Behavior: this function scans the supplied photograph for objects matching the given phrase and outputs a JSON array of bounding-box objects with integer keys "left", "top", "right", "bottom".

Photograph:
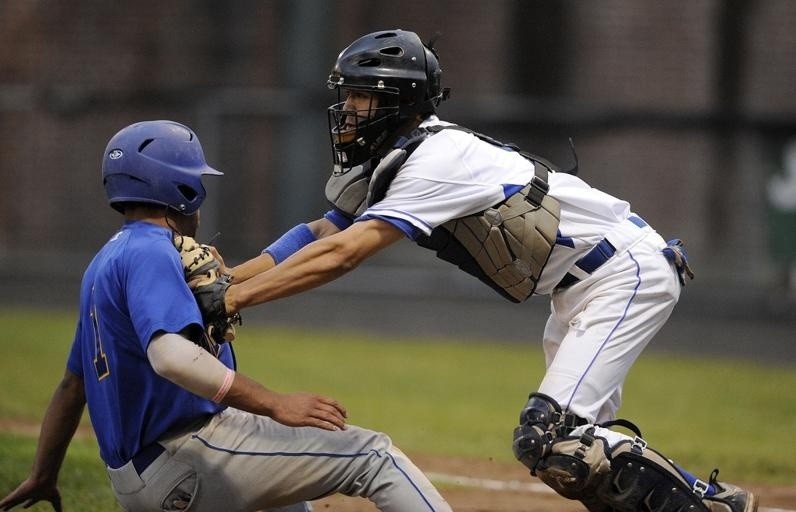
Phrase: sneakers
[{"left": 710, "top": 480, "right": 759, "bottom": 511}]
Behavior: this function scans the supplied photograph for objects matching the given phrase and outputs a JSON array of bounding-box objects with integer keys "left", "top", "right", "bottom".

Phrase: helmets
[
  {"left": 101, "top": 119, "right": 224, "bottom": 217},
  {"left": 326, "top": 28, "right": 441, "bottom": 177}
]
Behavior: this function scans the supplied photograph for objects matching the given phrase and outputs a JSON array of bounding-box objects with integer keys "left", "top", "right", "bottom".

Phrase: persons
[
  {"left": 172, "top": 27, "right": 759, "bottom": 511},
  {"left": 0, "top": 119, "right": 455, "bottom": 512}
]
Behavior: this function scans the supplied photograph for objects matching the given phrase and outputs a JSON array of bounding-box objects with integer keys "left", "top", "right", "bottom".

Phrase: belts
[
  {"left": 131, "top": 440, "right": 167, "bottom": 475},
  {"left": 554, "top": 216, "right": 646, "bottom": 287}
]
